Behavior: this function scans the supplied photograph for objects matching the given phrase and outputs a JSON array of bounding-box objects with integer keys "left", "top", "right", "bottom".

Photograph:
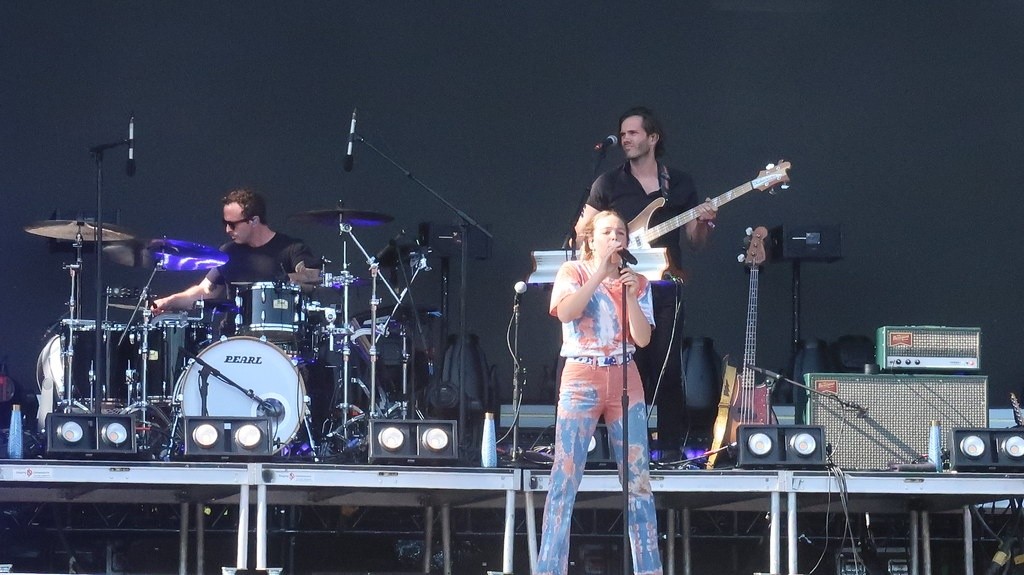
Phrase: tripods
[
  {"left": 54, "top": 229, "right": 185, "bottom": 459},
  {"left": 316, "top": 216, "right": 425, "bottom": 441}
]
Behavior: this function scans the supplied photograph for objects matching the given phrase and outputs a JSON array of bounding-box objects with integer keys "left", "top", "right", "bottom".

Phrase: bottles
[
  {"left": 928, "top": 419, "right": 943, "bottom": 473},
  {"left": 6, "top": 405, "right": 23, "bottom": 459},
  {"left": 481, "top": 413, "right": 498, "bottom": 468}
]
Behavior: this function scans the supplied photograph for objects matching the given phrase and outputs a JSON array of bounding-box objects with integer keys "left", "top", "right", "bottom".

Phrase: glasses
[{"left": 223, "top": 216, "right": 252, "bottom": 231}]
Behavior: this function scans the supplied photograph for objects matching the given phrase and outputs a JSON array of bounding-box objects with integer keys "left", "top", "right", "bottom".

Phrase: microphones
[
  {"left": 126, "top": 115, "right": 135, "bottom": 177},
  {"left": 594, "top": 135, "right": 618, "bottom": 150},
  {"left": 344, "top": 108, "right": 357, "bottom": 172},
  {"left": 618, "top": 248, "right": 638, "bottom": 265},
  {"left": 856, "top": 406, "right": 869, "bottom": 418},
  {"left": 373, "top": 229, "right": 404, "bottom": 263},
  {"left": 513, "top": 281, "right": 527, "bottom": 310},
  {"left": 254, "top": 395, "right": 281, "bottom": 417}
]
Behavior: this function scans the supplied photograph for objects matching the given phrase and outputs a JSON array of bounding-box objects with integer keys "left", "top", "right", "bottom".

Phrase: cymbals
[
  {"left": 285, "top": 208, "right": 396, "bottom": 227},
  {"left": 23, "top": 219, "right": 229, "bottom": 271},
  {"left": 303, "top": 277, "right": 381, "bottom": 287}
]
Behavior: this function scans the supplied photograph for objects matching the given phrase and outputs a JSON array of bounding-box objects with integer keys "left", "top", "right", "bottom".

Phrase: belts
[{"left": 570, "top": 354, "right": 634, "bottom": 366}]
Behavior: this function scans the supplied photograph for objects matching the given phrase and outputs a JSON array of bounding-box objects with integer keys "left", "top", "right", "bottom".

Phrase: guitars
[
  {"left": 704, "top": 226, "right": 780, "bottom": 469},
  {"left": 625, "top": 158, "right": 793, "bottom": 250}
]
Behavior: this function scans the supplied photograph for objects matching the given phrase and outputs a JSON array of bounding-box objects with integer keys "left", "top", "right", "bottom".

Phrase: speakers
[{"left": 803, "top": 373, "right": 990, "bottom": 471}]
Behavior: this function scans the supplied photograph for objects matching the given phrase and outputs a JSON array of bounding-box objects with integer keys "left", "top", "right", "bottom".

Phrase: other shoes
[{"left": 655, "top": 450, "right": 683, "bottom": 467}]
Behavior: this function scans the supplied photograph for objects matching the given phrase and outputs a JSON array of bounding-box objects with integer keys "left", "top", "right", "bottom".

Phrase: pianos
[{"left": 525, "top": 245, "right": 689, "bottom": 402}]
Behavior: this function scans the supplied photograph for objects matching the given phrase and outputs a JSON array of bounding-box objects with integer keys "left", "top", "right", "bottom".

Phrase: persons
[
  {"left": 150, "top": 189, "right": 322, "bottom": 457},
  {"left": 534, "top": 210, "right": 663, "bottom": 575},
  {"left": 569, "top": 106, "right": 718, "bottom": 462}
]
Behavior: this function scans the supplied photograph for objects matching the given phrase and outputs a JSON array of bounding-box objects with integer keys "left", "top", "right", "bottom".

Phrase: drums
[{"left": 36, "top": 280, "right": 357, "bottom": 459}]
